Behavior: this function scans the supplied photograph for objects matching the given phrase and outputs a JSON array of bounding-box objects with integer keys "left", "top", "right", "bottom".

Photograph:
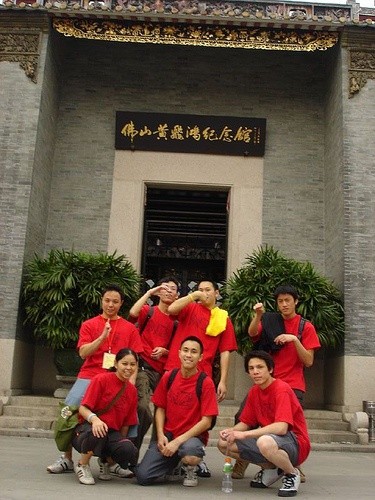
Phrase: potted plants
[{"left": 21, "top": 243, "right": 144, "bottom": 375}]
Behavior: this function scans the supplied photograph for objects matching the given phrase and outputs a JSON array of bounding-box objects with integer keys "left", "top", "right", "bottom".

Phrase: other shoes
[
  {"left": 297, "top": 466, "right": 305, "bottom": 482},
  {"left": 232, "top": 459, "right": 249, "bottom": 478}
]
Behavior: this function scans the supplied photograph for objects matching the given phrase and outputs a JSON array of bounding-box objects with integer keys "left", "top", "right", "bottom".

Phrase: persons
[
  {"left": 46, "top": 283, "right": 166, "bottom": 480},
  {"left": 74, "top": 348, "right": 139, "bottom": 486},
  {"left": 167, "top": 277, "right": 238, "bottom": 478},
  {"left": 134, "top": 335, "right": 219, "bottom": 487},
  {"left": 129, "top": 276, "right": 180, "bottom": 478},
  {"left": 231, "top": 284, "right": 321, "bottom": 477},
  {"left": 216, "top": 350, "right": 311, "bottom": 497}
]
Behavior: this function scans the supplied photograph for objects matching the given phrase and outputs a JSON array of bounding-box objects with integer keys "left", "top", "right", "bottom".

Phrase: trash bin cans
[{"left": 363, "top": 401, "right": 375, "bottom": 444}]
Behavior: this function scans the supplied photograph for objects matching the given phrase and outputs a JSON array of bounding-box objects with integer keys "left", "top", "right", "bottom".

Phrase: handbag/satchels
[
  {"left": 72, "top": 423, "right": 98, "bottom": 453},
  {"left": 54, "top": 400, "right": 81, "bottom": 451}
]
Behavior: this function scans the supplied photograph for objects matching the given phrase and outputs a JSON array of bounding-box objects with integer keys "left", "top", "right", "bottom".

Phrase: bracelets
[
  {"left": 189, "top": 293, "right": 194, "bottom": 302},
  {"left": 87, "top": 413, "right": 96, "bottom": 423}
]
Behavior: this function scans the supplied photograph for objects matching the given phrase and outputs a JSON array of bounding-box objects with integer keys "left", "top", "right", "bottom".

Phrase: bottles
[{"left": 221, "top": 458, "right": 234, "bottom": 494}]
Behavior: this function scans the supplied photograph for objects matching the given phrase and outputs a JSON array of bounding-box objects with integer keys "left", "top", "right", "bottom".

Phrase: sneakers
[
  {"left": 250, "top": 465, "right": 286, "bottom": 487},
  {"left": 76, "top": 461, "right": 95, "bottom": 484},
  {"left": 278, "top": 468, "right": 300, "bottom": 497},
  {"left": 197, "top": 461, "right": 211, "bottom": 477},
  {"left": 97, "top": 457, "right": 111, "bottom": 480},
  {"left": 109, "top": 463, "right": 133, "bottom": 477},
  {"left": 183, "top": 465, "right": 198, "bottom": 486},
  {"left": 47, "top": 456, "right": 75, "bottom": 473}
]
